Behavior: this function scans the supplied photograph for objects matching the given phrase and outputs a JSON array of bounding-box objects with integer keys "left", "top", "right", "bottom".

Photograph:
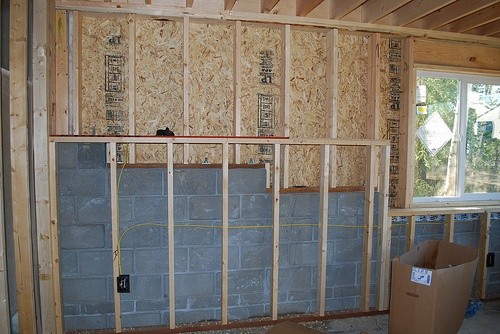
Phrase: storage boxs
[{"left": 385, "top": 239, "right": 480, "bottom": 334}]
